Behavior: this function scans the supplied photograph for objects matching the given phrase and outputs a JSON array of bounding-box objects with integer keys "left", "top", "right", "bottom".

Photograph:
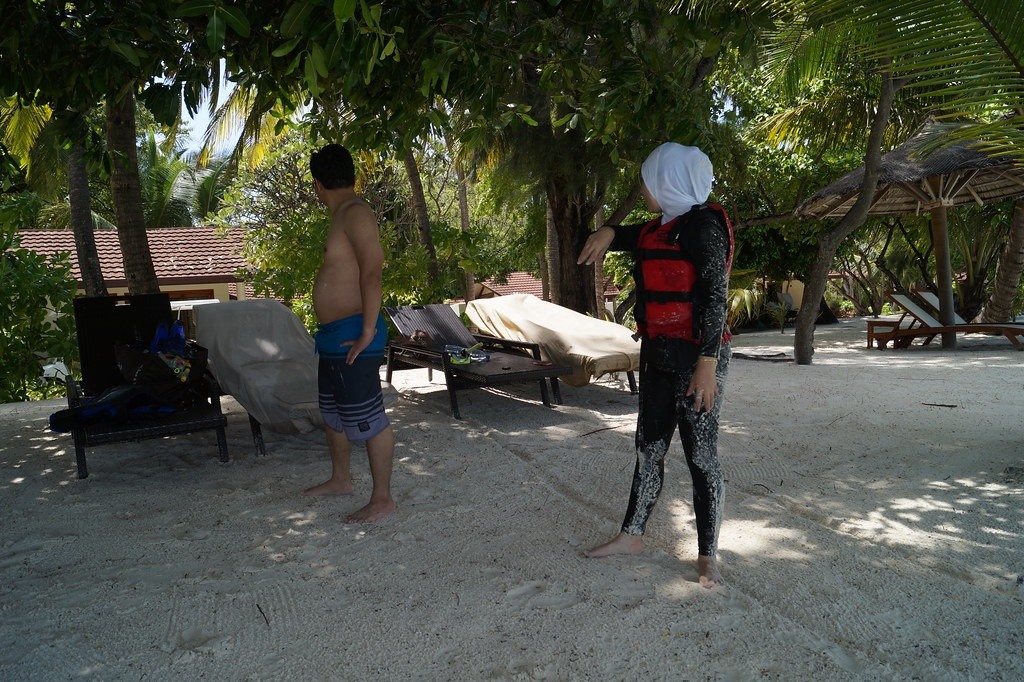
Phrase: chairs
[
  {"left": 191, "top": 299, "right": 398, "bottom": 457},
  {"left": 384, "top": 303, "right": 574, "bottom": 420},
  {"left": 463, "top": 292, "right": 642, "bottom": 405},
  {"left": 65, "top": 293, "right": 229, "bottom": 478},
  {"left": 874, "top": 292, "right": 1024, "bottom": 351},
  {"left": 912, "top": 288, "right": 1024, "bottom": 346}
]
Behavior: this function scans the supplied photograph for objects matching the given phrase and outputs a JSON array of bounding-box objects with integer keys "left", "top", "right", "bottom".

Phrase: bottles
[{"left": 445, "top": 345, "right": 491, "bottom": 362}]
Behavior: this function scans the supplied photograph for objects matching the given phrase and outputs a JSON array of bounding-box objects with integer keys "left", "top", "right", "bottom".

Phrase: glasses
[{"left": 312, "top": 179, "right": 316, "bottom": 187}]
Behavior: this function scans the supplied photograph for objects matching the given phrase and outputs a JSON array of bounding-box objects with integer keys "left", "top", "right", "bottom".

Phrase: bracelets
[{"left": 699, "top": 359, "right": 718, "bottom": 362}]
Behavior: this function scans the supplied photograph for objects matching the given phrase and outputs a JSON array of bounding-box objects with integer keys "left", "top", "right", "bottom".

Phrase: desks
[{"left": 866, "top": 320, "right": 901, "bottom": 349}]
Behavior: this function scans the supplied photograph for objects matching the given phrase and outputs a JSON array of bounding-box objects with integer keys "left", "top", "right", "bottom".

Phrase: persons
[
  {"left": 577, "top": 142, "right": 734, "bottom": 589},
  {"left": 301, "top": 143, "right": 394, "bottom": 523}
]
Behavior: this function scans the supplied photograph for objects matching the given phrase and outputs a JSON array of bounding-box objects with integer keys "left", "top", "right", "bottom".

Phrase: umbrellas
[{"left": 792, "top": 115, "right": 1024, "bottom": 351}]
